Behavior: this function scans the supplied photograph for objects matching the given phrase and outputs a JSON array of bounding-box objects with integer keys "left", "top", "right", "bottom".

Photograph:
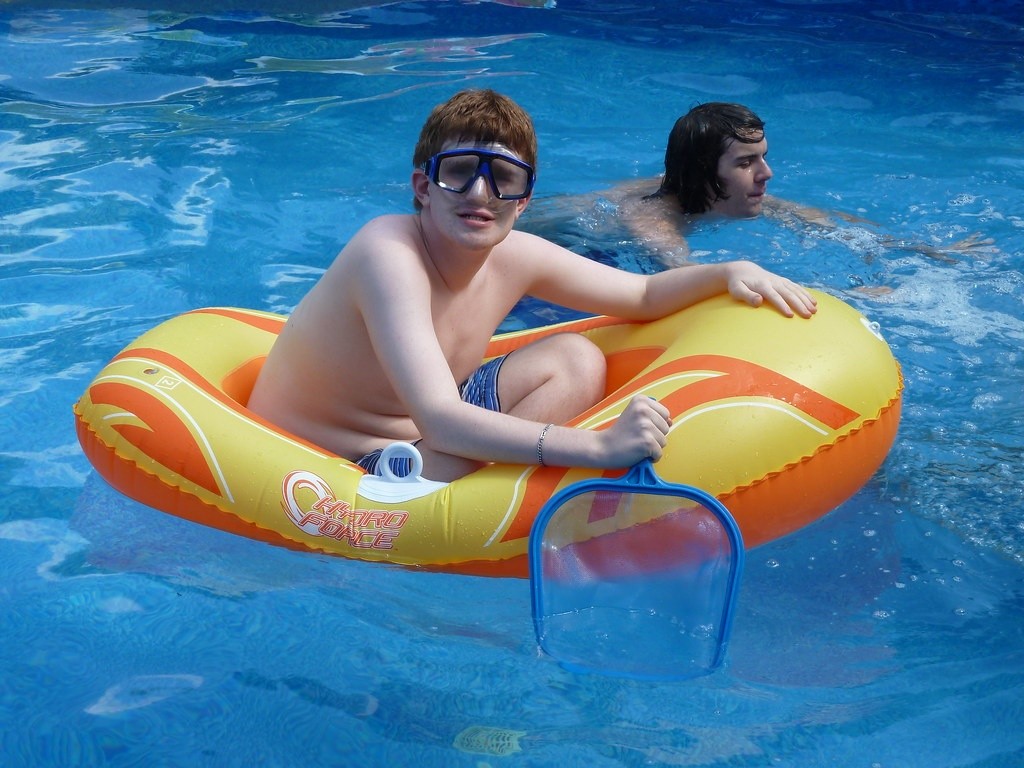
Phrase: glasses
[{"left": 418, "top": 149, "right": 534, "bottom": 200}]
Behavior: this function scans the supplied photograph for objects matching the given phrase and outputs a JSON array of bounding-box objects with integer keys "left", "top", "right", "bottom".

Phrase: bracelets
[{"left": 535, "top": 422, "right": 555, "bottom": 468}]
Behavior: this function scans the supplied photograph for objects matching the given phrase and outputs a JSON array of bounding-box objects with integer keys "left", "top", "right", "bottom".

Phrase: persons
[
  {"left": 510, "top": 102, "right": 999, "bottom": 300},
  {"left": 238, "top": 86, "right": 819, "bottom": 483}
]
